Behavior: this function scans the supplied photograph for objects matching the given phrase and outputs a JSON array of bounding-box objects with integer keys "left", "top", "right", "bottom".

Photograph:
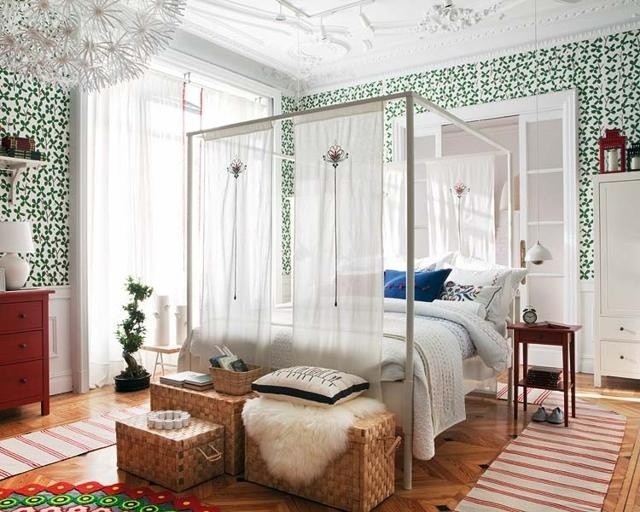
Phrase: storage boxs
[
  {"left": 116, "top": 411, "right": 225, "bottom": 493},
  {"left": 244, "top": 409, "right": 397, "bottom": 511},
  {"left": 149, "top": 381, "right": 258, "bottom": 477}
]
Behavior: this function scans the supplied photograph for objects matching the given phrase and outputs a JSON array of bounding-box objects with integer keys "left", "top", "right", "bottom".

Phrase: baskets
[{"left": 207, "top": 361, "right": 264, "bottom": 396}]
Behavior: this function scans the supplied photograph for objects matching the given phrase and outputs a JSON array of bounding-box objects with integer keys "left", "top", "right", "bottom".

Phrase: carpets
[
  {"left": 1, "top": 481, "right": 222, "bottom": 512},
  {"left": 0, "top": 401, "right": 150, "bottom": 481},
  {"left": 449, "top": 380, "right": 629, "bottom": 512}
]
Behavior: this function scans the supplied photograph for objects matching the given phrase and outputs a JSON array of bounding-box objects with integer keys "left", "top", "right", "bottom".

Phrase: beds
[{"left": 175, "top": 91, "right": 527, "bottom": 491}]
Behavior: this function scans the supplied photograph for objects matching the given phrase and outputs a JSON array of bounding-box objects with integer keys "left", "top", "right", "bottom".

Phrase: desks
[
  {"left": 505, "top": 321, "right": 583, "bottom": 427},
  {"left": 139, "top": 344, "right": 181, "bottom": 376}
]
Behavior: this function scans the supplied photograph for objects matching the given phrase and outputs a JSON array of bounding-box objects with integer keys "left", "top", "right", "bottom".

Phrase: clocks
[{"left": 523, "top": 305, "right": 537, "bottom": 324}]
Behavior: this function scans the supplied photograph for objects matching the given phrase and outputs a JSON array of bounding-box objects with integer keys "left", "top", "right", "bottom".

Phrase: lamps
[
  {"left": 523, "top": 1, "right": 554, "bottom": 266},
  {"left": 0, "top": 221, "right": 36, "bottom": 291}
]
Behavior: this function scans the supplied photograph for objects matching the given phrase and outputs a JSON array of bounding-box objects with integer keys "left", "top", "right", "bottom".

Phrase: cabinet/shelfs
[
  {"left": 0, "top": 288, "right": 55, "bottom": 417},
  {"left": 592, "top": 170, "right": 640, "bottom": 388}
]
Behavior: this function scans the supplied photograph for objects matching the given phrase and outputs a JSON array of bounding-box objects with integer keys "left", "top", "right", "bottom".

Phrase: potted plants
[{"left": 112, "top": 276, "right": 154, "bottom": 393}]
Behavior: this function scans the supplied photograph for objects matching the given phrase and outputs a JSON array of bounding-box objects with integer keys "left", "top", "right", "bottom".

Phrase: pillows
[
  {"left": 382, "top": 249, "right": 528, "bottom": 318},
  {"left": 251, "top": 366, "right": 369, "bottom": 408}
]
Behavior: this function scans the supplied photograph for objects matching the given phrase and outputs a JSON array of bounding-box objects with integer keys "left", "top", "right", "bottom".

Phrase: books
[
  {"left": 160, "top": 371, "right": 213, "bottom": 391},
  {"left": 209, "top": 354, "right": 248, "bottom": 372}
]
